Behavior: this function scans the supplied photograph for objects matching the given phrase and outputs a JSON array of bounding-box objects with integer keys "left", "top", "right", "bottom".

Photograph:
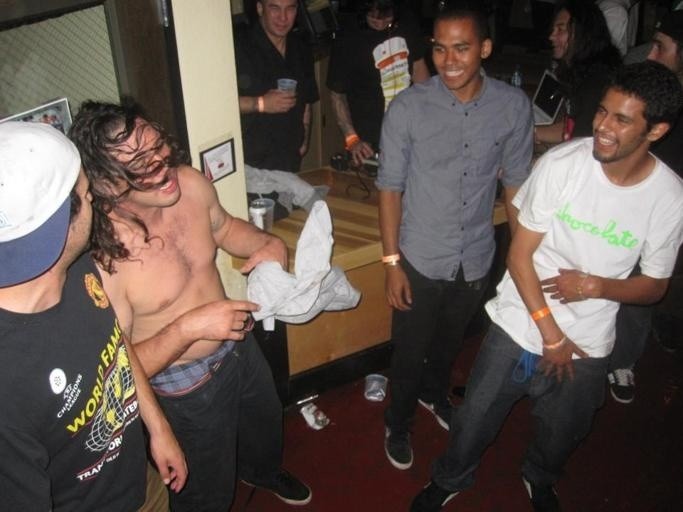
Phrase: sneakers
[
  {"left": 652, "top": 318, "right": 683, "bottom": 355},
  {"left": 409, "top": 476, "right": 461, "bottom": 512},
  {"left": 383, "top": 410, "right": 415, "bottom": 470},
  {"left": 608, "top": 368, "right": 637, "bottom": 403},
  {"left": 419, "top": 394, "right": 455, "bottom": 434},
  {"left": 522, "top": 471, "right": 562, "bottom": 512},
  {"left": 238, "top": 465, "right": 312, "bottom": 506}
]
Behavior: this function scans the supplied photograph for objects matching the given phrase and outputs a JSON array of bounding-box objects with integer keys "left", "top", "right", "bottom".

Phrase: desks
[{"left": 262, "top": 162, "right": 508, "bottom": 401}]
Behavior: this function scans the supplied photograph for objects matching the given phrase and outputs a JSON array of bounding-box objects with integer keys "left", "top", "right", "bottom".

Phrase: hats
[
  {"left": 656, "top": 10, "right": 683, "bottom": 43},
  {"left": 0, "top": 120, "right": 81, "bottom": 291}
]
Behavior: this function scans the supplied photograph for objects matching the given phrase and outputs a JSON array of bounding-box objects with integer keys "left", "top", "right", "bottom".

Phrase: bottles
[{"left": 510, "top": 72, "right": 522, "bottom": 88}]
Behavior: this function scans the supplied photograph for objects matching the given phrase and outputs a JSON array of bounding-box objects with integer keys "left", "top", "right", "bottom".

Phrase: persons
[
  {"left": 374, "top": 4, "right": 533, "bottom": 467},
  {"left": 230, "top": 2, "right": 682, "bottom": 225},
  {"left": 0, "top": 121, "right": 187, "bottom": 509},
  {"left": 66, "top": 97, "right": 311, "bottom": 510},
  {"left": 401, "top": 62, "right": 682, "bottom": 509}
]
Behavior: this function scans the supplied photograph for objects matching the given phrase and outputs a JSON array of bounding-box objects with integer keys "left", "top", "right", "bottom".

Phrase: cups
[
  {"left": 249, "top": 200, "right": 275, "bottom": 232},
  {"left": 277, "top": 78, "right": 298, "bottom": 92}
]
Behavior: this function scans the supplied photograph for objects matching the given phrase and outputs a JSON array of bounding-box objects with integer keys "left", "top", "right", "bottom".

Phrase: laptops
[{"left": 530, "top": 70, "right": 566, "bottom": 126}]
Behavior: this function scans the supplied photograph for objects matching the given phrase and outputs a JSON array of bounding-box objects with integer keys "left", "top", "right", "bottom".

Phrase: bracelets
[
  {"left": 380, "top": 253, "right": 402, "bottom": 265},
  {"left": 539, "top": 334, "right": 566, "bottom": 353},
  {"left": 574, "top": 271, "right": 594, "bottom": 302},
  {"left": 528, "top": 304, "right": 552, "bottom": 325}
]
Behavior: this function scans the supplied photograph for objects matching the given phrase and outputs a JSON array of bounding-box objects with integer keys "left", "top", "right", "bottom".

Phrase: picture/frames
[
  {"left": 198, "top": 137, "right": 236, "bottom": 184},
  {"left": 0, "top": 97, "right": 72, "bottom": 136}
]
